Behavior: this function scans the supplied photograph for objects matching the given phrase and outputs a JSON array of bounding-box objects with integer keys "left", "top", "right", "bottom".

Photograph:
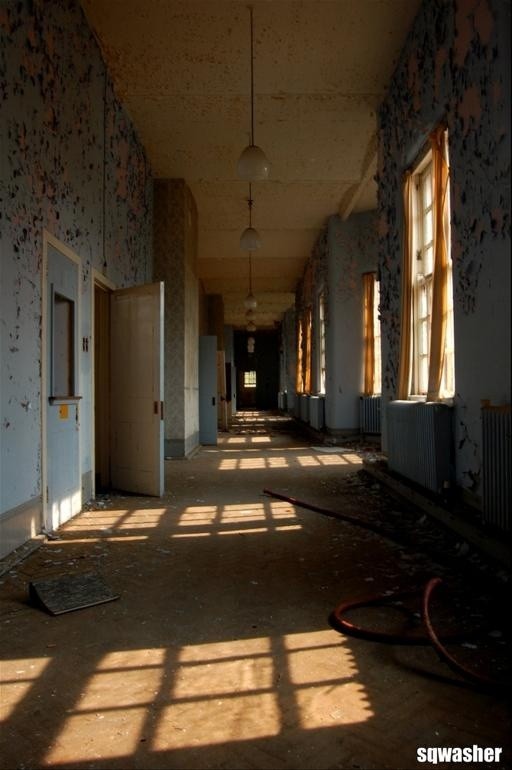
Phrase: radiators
[
  {"left": 385, "top": 401, "right": 455, "bottom": 498},
  {"left": 277, "top": 392, "right": 323, "bottom": 432},
  {"left": 481, "top": 404, "right": 512, "bottom": 539},
  {"left": 359, "top": 396, "right": 382, "bottom": 443}
]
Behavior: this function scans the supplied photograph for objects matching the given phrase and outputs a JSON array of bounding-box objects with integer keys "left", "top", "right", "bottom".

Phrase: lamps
[{"left": 234, "top": 1, "right": 273, "bottom": 352}]
